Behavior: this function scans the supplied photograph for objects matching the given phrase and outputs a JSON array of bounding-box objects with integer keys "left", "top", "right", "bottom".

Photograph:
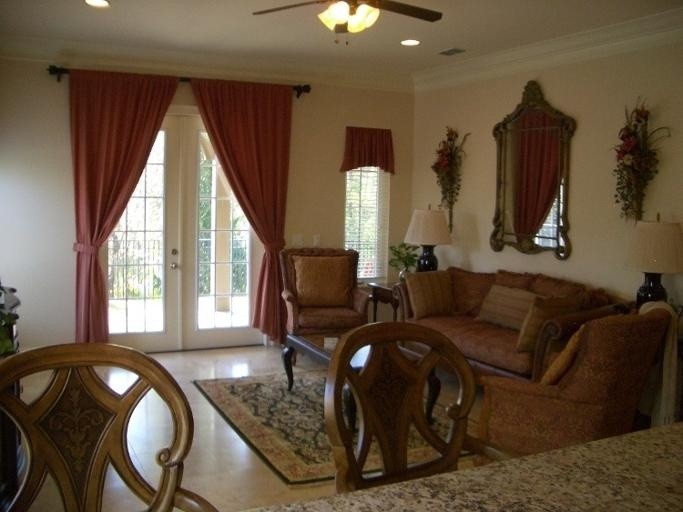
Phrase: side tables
[{"left": 367, "top": 279, "right": 399, "bottom": 322}]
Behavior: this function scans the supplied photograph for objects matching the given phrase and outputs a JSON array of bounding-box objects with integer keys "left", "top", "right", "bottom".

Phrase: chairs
[{"left": 0, "top": 340, "right": 220, "bottom": 511}]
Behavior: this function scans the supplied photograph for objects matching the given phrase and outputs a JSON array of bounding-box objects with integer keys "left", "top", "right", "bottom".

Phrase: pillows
[
  {"left": 528, "top": 271, "right": 585, "bottom": 296},
  {"left": 446, "top": 265, "right": 495, "bottom": 317},
  {"left": 496, "top": 267, "right": 539, "bottom": 289},
  {"left": 514, "top": 295, "right": 584, "bottom": 352},
  {"left": 287, "top": 252, "right": 356, "bottom": 310},
  {"left": 471, "top": 284, "right": 548, "bottom": 332},
  {"left": 404, "top": 269, "right": 454, "bottom": 321}
]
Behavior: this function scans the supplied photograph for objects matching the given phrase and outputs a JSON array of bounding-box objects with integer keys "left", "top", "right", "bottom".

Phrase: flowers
[
  {"left": 427, "top": 124, "right": 473, "bottom": 234},
  {"left": 605, "top": 93, "right": 672, "bottom": 230}
]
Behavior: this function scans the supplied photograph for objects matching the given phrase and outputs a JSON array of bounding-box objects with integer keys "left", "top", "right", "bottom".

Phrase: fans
[{"left": 249, "top": 1, "right": 444, "bottom": 25}]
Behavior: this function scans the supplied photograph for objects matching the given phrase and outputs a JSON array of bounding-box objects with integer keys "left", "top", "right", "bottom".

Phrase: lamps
[
  {"left": 403, "top": 203, "right": 452, "bottom": 273},
  {"left": 315, "top": 1, "right": 380, "bottom": 49},
  {"left": 621, "top": 212, "right": 683, "bottom": 314}
]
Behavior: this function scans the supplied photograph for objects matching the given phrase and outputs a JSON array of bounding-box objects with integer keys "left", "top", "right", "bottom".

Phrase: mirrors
[{"left": 488, "top": 80, "right": 577, "bottom": 261}]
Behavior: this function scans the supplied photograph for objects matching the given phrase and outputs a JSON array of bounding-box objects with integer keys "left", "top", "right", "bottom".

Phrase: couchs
[
  {"left": 276, "top": 246, "right": 374, "bottom": 367},
  {"left": 472, "top": 306, "right": 671, "bottom": 467},
  {"left": 389, "top": 264, "right": 637, "bottom": 383}
]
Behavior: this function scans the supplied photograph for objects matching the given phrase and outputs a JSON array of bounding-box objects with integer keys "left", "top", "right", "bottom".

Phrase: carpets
[{"left": 189, "top": 366, "right": 480, "bottom": 491}]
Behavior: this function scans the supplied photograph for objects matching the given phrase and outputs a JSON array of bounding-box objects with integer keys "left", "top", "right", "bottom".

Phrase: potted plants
[{"left": 387, "top": 241, "right": 420, "bottom": 282}]
